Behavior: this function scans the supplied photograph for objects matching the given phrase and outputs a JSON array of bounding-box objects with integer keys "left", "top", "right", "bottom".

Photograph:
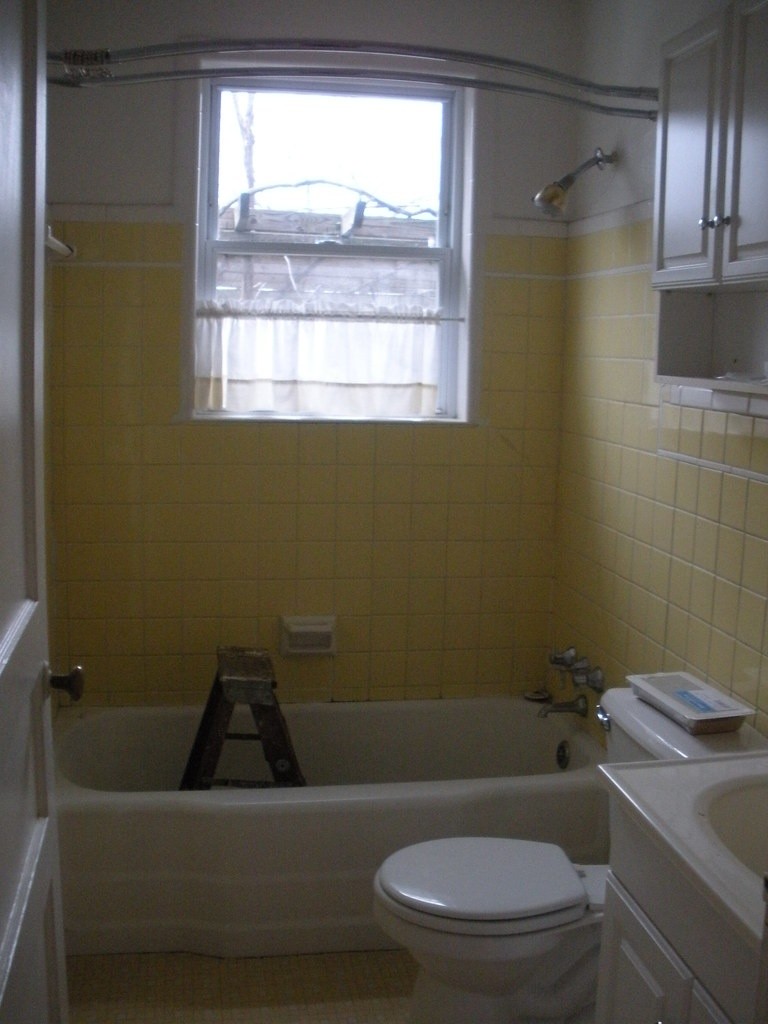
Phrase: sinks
[{"left": 688, "top": 769, "right": 768, "bottom": 910}]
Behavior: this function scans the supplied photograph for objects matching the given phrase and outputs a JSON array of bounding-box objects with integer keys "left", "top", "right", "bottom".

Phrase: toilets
[{"left": 371, "top": 684, "right": 767, "bottom": 1023}]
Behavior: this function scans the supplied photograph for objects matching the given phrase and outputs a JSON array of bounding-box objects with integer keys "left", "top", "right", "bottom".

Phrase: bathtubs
[{"left": 54, "top": 693, "right": 607, "bottom": 961}]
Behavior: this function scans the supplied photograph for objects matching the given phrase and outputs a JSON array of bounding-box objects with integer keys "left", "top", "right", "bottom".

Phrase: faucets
[{"left": 537, "top": 695, "right": 588, "bottom": 719}]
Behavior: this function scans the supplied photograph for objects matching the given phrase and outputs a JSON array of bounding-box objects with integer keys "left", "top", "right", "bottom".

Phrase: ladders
[{"left": 174, "top": 646, "right": 308, "bottom": 791}]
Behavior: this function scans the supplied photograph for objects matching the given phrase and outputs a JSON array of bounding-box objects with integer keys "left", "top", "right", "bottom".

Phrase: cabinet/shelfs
[
  {"left": 649, "top": 0, "right": 767, "bottom": 397},
  {"left": 592, "top": 790, "right": 768, "bottom": 1024}
]
